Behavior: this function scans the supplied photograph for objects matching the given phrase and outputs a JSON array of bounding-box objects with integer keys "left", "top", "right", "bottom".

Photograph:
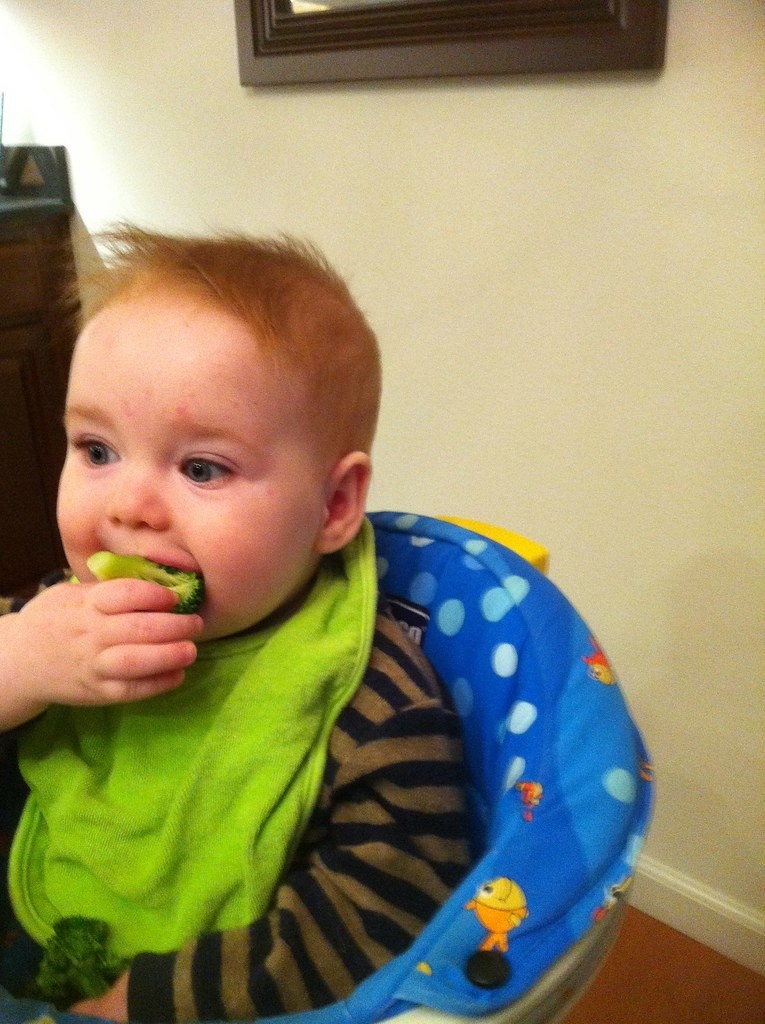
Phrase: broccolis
[
  {"left": 39, "top": 916, "right": 117, "bottom": 1003},
  {"left": 68, "top": 550, "right": 205, "bottom": 615}
]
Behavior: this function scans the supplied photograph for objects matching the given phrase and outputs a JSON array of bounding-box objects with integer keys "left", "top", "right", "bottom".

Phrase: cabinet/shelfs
[{"left": 0, "top": 182, "right": 82, "bottom": 597}]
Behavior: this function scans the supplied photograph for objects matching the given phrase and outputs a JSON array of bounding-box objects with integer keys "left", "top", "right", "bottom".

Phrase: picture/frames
[{"left": 234, "top": 0, "right": 668, "bottom": 86}]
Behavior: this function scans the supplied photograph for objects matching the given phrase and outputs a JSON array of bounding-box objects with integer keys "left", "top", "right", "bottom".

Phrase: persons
[{"left": 0, "top": 232, "right": 476, "bottom": 1024}]
